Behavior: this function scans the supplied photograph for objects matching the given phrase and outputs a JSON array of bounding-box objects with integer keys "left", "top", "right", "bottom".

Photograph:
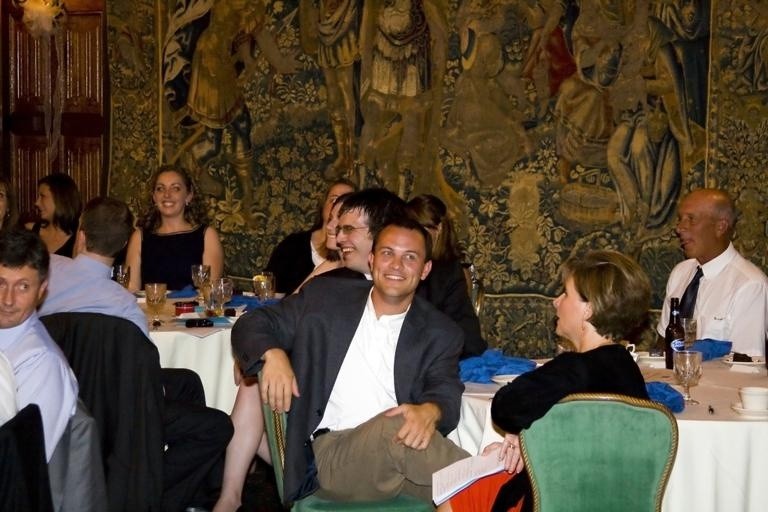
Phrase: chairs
[
  {"left": 256, "top": 370, "right": 433, "bottom": 512},
  {"left": 0, "top": 403, "right": 55, "bottom": 512},
  {"left": 37, "top": 312, "right": 235, "bottom": 512},
  {"left": 517, "top": 393, "right": 677, "bottom": 512}
]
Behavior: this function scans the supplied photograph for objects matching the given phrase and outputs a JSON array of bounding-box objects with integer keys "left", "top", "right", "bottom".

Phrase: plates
[
  {"left": 721, "top": 359, "right": 766, "bottom": 365},
  {"left": 228, "top": 316, "right": 239, "bottom": 321},
  {"left": 640, "top": 355, "right": 666, "bottom": 365},
  {"left": 731, "top": 405, "right": 768, "bottom": 416},
  {"left": 490, "top": 374, "right": 519, "bottom": 381}
]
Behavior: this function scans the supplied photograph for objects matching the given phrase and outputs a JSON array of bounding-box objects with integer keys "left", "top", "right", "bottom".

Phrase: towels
[
  {"left": 167, "top": 285, "right": 199, "bottom": 298},
  {"left": 224, "top": 296, "right": 257, "bottom": 306},
  {"left": 687, "top": 338, "right": 732, "bottom": 362},
  {"left": 481, "top": 350, "right": 537, "bottom": 375},
  {"left": 458, "top": 357, "right": 498, "bottom": 383},
  {"left": 645, "top": 381, "right": 685, "bottom": 413},
  {"left": 243, "top": 299, "right": 278, "bottom": 311}
]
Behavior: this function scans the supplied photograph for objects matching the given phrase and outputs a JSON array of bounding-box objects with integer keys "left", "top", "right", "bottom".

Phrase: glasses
[{"left": 335, "top": 225, "right": 369, "bottom": 235}]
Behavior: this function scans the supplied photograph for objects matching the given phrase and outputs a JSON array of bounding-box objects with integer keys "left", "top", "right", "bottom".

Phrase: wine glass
[
  {"left": 252, "top": 272, "right": 272, "bottom": 300},
  {"left": 146, "top": 283, "right": 166, "bottom": 326},
  {"left": 192, "top": 265, "right": 211, "bottom": 302},
  {"left": 116, "top": 265, "right": 130, "bottom": 287},
  {"left": 681, "top": 318, "right": 697, "bottom": 349},
  {"left": 673, "top": 352, "right": 703, "bottom": 406},
  {"left": 206, "top": 284, "right": 223, "bottom": 317}
]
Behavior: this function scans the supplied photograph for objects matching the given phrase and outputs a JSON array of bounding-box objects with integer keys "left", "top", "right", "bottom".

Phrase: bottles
[{"left": 664, "top": 295, "right": 687, "bottom": 370}]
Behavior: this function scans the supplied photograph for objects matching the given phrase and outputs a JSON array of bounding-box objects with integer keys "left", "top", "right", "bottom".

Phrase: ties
[{"left": 679, "top": 266, "right": 704, "bottom": 318}]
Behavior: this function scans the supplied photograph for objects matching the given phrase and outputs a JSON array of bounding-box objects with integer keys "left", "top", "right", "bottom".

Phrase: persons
[
  {"left": 654, "top": 188, "right": 765, "bottom": 356},
  {"left": 18, "top": 172, "right": 82, "bottom": 255},
  {"left": 0, "top": 224, "right": 80, "bottom": 467},
  {"left": 230, "top": 215, "right": 475, "bottom": 512},
  {"left": 296, "top": 189, "right": 408, "bottom": 295},
  {"left": 32, "top": 195, "right": 150, "bottom": 350},
  {"left": 262, "top": 180, "right": 356, "bottom": 292},
  {"left": 124, "top": 167, "right": 222, "bottom": 306},
  {"left": 433, "top": 251, "right": 648, "bottom": 512},
  {"left": 212, "top": 189, "right": 355, "bottom": 511},
  {"left": 404, "top": 195, "right": 487, "bottom": 358}
]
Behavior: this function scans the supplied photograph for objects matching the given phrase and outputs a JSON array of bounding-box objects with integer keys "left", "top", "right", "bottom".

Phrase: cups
[
  {"left": 740, "top": 387, "right": 768, "bottom": 411},
  {"left": 215, "top": 278, "right": 233, "bottom": 303}
]
